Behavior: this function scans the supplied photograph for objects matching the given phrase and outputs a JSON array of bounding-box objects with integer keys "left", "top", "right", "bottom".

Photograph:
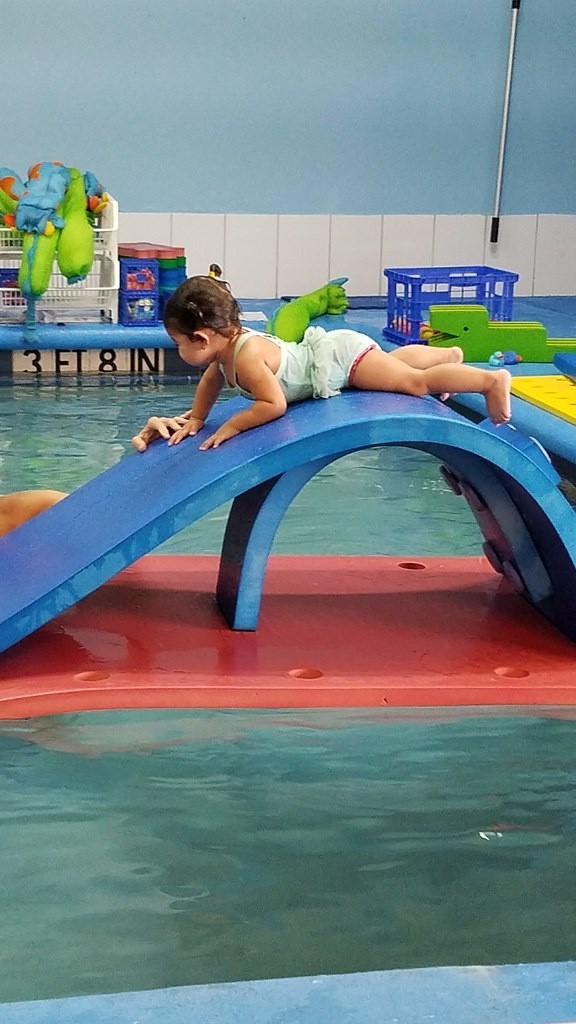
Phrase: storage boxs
[
  {"left": 382, "top": 265, "right": 519, "bottom": 346},
  {"left": 238, "top": 311, "right": 269, "bottom": 333},
  {"left": 0, "top": 192, "right": 161, "bottom": 328}
]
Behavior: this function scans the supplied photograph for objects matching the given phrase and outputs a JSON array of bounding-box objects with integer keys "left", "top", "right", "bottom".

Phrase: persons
[{"left": 162, "top": 264, "right": 512, "bottom": 451}]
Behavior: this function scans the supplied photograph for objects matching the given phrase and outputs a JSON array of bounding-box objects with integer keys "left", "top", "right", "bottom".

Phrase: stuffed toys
[
  {"left": 265, "top": 277, "right": 350, "bottom": 344},
  {"left": 0, "top": 161, "right": 110, "bottom": 299}
]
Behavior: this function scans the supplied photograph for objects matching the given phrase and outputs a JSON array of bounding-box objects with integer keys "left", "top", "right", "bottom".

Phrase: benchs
[{"left": 0, "top": 321, "right": 199, "bottom": 373}]
